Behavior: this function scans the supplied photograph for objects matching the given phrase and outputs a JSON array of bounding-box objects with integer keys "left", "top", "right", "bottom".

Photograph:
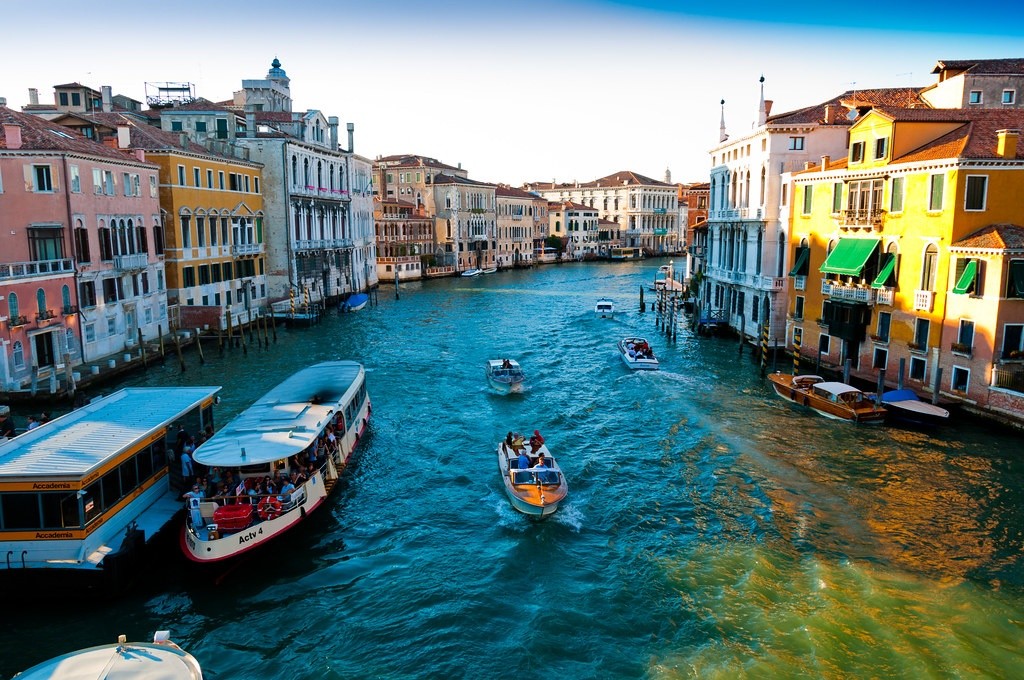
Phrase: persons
[
  {"left": 501, "top": 358, "right": 513, "bottom": 371},
  {"left": 533, "top": 452, "right": 551, "bottom": 480},
  {"left": 507, "top": 430, "right": 544, "bottom": 455},
  {"left": 628, "top": 340, "right": 653, "bottom": 358},
  {"left": 28, "top": 415, "right": 40, "bottom": 429},
  {"left": 518, "top": 449, "right": 530, "bottom": 469},
  {"left": 168, "top": 413, "right": 343, "bottom": 499},
  {"left": 40, "top": 412, "right": 51, "bottom": 424},
  {"left": 0, "top": 415, "right": 15, "bottom": 437}
]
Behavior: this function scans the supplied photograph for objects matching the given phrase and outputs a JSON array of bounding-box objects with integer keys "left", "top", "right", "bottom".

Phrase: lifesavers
[{"left": 257, "top": 497, "right": 282, "bottom": 520}]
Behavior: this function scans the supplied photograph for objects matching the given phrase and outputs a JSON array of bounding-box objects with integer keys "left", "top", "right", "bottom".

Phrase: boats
[
  {"left": 481, "top": 268, "right": 498, "bottom": 274},
  {"left": 11, "top": 629, "right": 202, "bottom": 680},
  {"left": 497, "top": 430, "right": 569, "bottom": 518},
  {"left": 610, "top": 248, "right": 643, "bottom": 259},
  {"left": 486, "top": 359, "right": 523, "bottom": 392},
  {"left": 861, "top": 388, "right": 950, "bottom": 419},
  {"left": 595, "top": 300, "right": 614, "bottom": 319},
  {"left": 178, "top": 359, "right": 373, "bottom": 564},
  {"left": 649, "top": 277, "right": 685, "bottom": 291},
  {"left": 339, "top": 293, "right": 369, "bottom": 311},
  {"left": 766, "top": 373, "right": 887, "bottom": 424},
  {"left": 462, "top": 268, "right": 482, "bottom": 276},
  {"left": 618, "top": 337, "right": 660, "bottom": 370},
  {"left": 0, "top": 383, "right": 220, "bottom": 569}
]
